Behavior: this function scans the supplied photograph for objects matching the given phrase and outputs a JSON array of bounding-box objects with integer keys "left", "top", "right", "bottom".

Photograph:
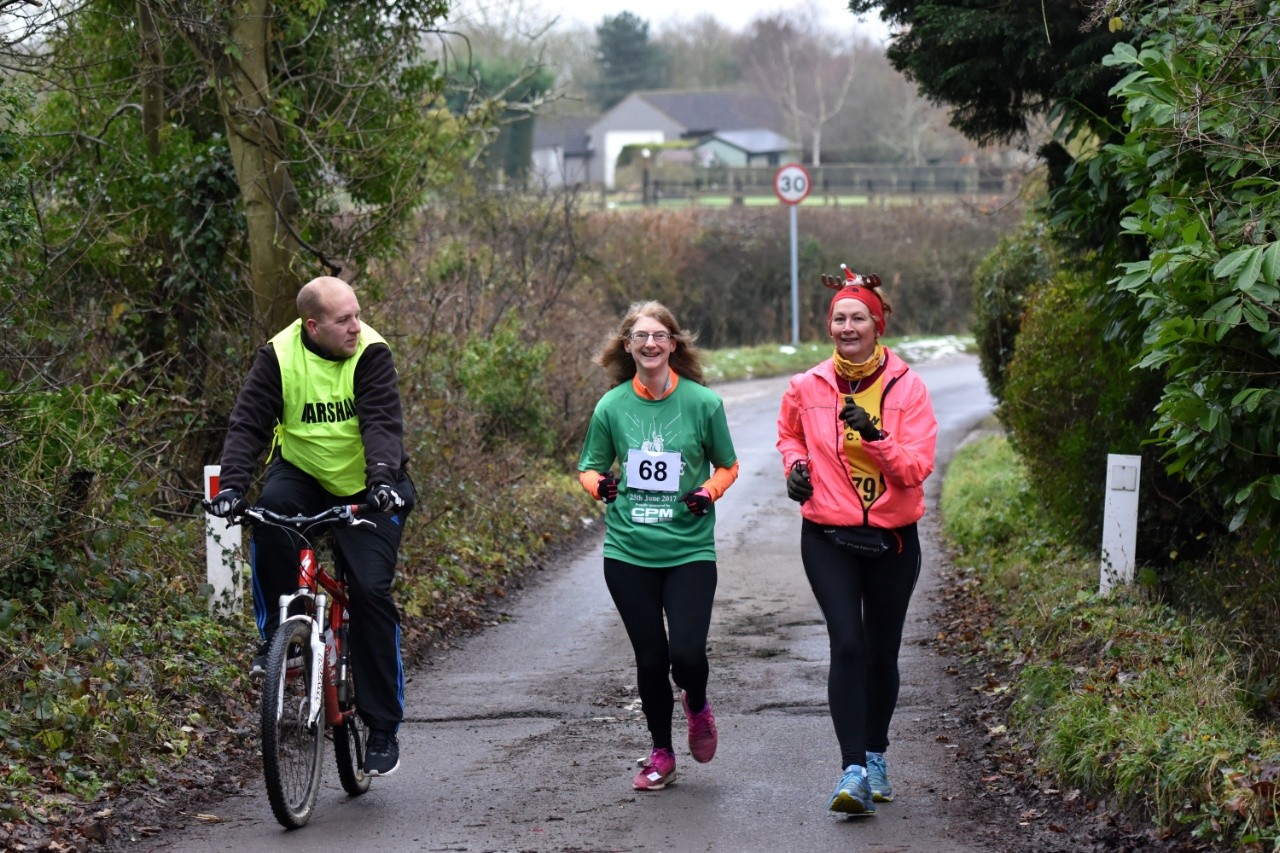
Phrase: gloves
[
  {"left": 676, "top": 486, "right": 711, "bottom": 517},
  {"left": 593, "top": 471, "right": 620, "bottom": 504},
  {"left": 369, "top": 482, "right": 408, "bottom": 518},
  {"left": 838, "top": 396, "right": 880, "bottom": 442},
  {"left": 787, "top": 462, "right": 816, "bottom": 502},
  {"left": 209, "top": 488, "right": 250, "bottom": 516}
]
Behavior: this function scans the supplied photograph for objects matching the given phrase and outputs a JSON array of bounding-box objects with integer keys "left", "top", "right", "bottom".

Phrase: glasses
[
  {"left": 795, "top": 459, "right": 812, "bottom": 477},
  {"left": 627, "top": 330, "right": 675, "bottom": 344}
]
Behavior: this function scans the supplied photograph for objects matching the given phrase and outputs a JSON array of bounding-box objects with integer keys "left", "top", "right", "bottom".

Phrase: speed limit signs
[{"left": 772, "top": 160, "right": 811, "bottom": 205}]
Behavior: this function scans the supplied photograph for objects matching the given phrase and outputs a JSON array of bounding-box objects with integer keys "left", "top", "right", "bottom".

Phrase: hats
[{"left": 820, "top": 263, "right": 887, "bottom": 336}]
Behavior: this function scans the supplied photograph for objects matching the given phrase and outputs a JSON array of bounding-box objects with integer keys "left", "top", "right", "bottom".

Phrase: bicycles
[{"left": 204, "top": 487, "right": 382, "bottom": 831}]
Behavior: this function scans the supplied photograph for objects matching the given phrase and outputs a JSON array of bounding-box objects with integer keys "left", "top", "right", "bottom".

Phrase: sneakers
[
  {"left": 829, "top": 764, "right": 879, "bottom": 815},
  {"left": 363, "top": 732, "right": 403, "bottom": 781},
  {"left": 680, "top": 689, "right": 718, "bottom": 764},
  {"left": 633, "top": 746, "right": 676, "bottom": 790},
  {"left": 865, "top": 750, "right": 895, "bottom": 802},
  {"left": 248, "top": 642, "right": 309, "bottom": 677}
]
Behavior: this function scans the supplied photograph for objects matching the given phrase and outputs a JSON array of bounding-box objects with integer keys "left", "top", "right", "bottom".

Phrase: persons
[
  {"left": 776, "top": 260, "right": 937, "bottom": 822},
  {"left": 212, "top": 275, "right": 414, "bottom": 776},
  {"left": 579, "top": 301, "right": 738, "bottom": 790}
]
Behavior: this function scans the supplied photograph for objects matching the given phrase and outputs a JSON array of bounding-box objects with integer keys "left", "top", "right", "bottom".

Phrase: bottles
[{"left": 320, "top": 617, "right": 338, "bottom": 688}]
